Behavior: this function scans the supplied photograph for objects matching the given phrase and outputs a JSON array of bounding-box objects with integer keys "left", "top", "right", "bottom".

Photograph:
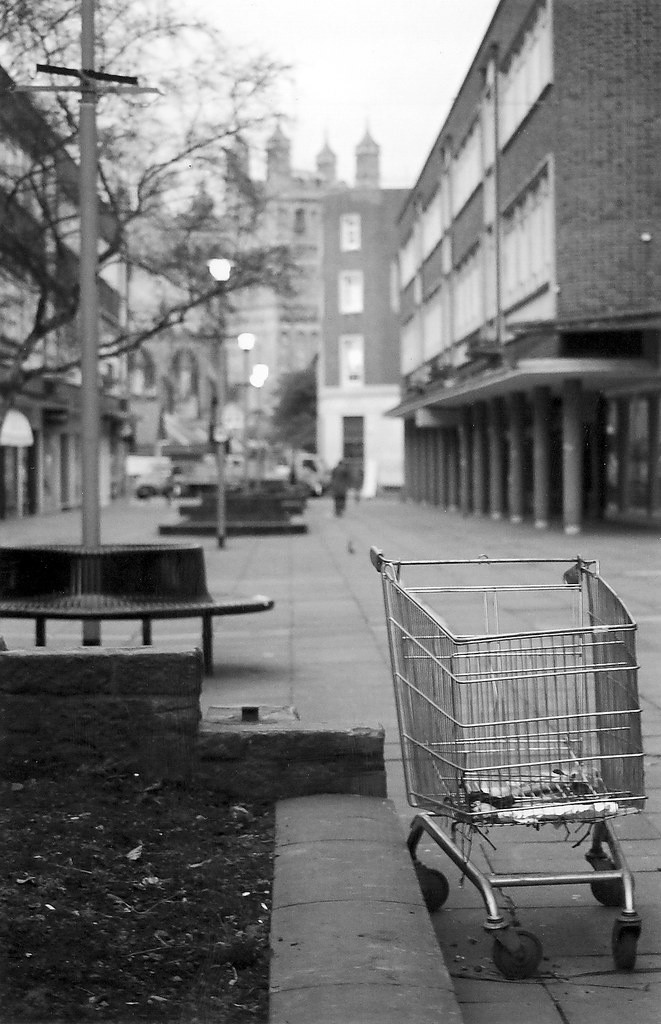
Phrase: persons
[{"left": 333, "top": 461, "right": 351, "bottom": 516}]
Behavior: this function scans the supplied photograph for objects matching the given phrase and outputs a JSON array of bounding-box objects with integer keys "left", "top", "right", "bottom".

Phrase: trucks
[{"left": 190, "top": 447, "right": 332, "bottom": 498}]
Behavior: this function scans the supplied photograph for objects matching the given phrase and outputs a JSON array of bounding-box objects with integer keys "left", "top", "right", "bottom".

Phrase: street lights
[{"left": 209, "top": 259, "right": 234, "bottom": 548}]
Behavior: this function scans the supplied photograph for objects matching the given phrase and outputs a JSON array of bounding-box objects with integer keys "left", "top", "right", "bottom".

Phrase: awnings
[{"left": 0, "top": 409, "right": 33, "bottom": 447}]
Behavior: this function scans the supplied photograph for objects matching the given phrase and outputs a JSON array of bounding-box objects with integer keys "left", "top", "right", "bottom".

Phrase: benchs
[{"left": 0, "top": 543, "right": 276, "bottom": 677}]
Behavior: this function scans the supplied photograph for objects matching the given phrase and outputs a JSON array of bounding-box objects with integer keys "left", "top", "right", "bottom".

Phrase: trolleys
[{"left": 368, "top": 545, "right": 647, "bottom": 980}]
